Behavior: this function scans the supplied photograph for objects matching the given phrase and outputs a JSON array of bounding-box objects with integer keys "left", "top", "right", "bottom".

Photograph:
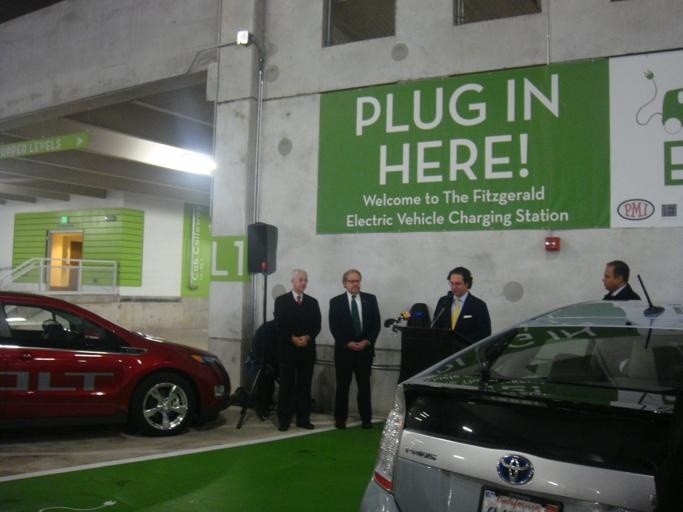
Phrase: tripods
[{"left": 237, "top": 283, "right": 280, "bottom": 427}]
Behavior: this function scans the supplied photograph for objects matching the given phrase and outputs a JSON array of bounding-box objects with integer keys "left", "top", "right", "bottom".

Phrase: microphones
[{"left": 401, "top": 311, "right": 423, "bottom": 321}]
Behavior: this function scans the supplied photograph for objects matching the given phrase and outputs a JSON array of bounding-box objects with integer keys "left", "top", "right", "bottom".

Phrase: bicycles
[{"left": 235, "top": 357, "right": 275, "bottom": 434}]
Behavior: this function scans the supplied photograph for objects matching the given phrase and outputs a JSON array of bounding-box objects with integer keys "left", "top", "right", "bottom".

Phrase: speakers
[{"left": 247, "top": 222, "right": 279, "bottom": 275}]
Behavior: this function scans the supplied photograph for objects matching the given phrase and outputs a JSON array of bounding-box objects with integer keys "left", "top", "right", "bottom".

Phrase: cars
[
  {"left": 0, "top": 289, "right": 238, "bottom": 443},
  {"left": 356, "top": 284, "right": 683, "bottom": 511}
]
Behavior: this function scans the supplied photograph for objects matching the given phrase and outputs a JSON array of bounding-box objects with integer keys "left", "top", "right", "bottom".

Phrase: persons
[
  {"left": 600, "top": 260, "right": 643, "bottom": 301},
  {"left": 431, "top": 266, "right": 492, "bottom": 341},
  {"left": 273, "top": 268, "right": 321, "bottom": 432},
  {"left": 245, "top": 317, "right": 277, "bottom": 419},
  {"left": 327, "top": 269, "right": 380, "bottom": 430}
]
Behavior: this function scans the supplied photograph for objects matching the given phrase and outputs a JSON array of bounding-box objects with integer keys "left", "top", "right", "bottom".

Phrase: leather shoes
[
  {"left": 278, "top": 422, "right": 289, "bottom": 431},
  {"left": 362, "top": 421, "right": 372, "bottom": 428},
  {"left": 296, "top": 424, "right": 314, "bottom": 429},
  {"left": 335, "top": 419, "right": 346, "bottom": 428}
]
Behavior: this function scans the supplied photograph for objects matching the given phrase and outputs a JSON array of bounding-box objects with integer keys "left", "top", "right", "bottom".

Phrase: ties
[
  {"left": 451, "top": 298, "right": 461, "bottom": 332},
  {"left": 296, "top": 296, "right": 301, "bottom": 304},
  {"left": 351, "top": 294, "right": 361, "bottom": 336}
]
